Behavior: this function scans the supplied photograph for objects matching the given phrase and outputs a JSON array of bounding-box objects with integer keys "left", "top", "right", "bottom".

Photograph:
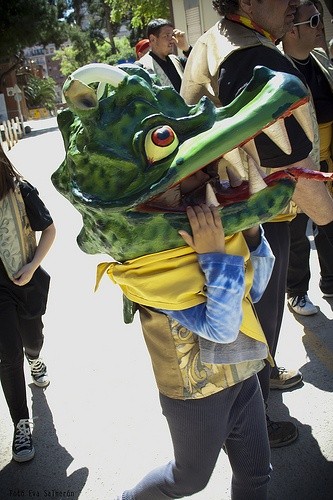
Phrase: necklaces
[{"left": 288, "top": 55, "right": 310, "bottom": 66}]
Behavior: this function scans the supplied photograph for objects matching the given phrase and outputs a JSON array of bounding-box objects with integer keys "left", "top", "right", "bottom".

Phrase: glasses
[
  {"left": 158, "top": 33, "right": 175, "bottom": 39},
  {"left": 294, "top": 13, "right": 321, "bottom": 29}
]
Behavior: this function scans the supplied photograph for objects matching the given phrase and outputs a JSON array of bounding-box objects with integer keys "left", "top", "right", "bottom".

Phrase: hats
[{"left": 136, "top": 39, "right": 149, "bottom": 54}]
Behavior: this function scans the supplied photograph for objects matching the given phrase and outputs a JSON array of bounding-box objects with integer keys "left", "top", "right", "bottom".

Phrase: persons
[
  {"left": 274, "top": 0, "right": 333, "bottom": 317},
  {"left": 119, "top": 167, "right": 276, "bottom": 500},
  {"left": 134, "top": 39, "right": 151, "bottom": 62},
  {"left": 178, "top": 0, "right": 333, "bottom": 450},
  {"left": 0, "top": 143, "right": 56, "bottom": 464},
  {"left": 136, "top": 18, "right": 192, "bottom": 94}
]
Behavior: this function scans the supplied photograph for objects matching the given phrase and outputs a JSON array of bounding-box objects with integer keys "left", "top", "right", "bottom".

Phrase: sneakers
[
  {"left": 323, "top": 293, "right": 333, "bottom": 298},
  {"left": 270, "top": 366, "right": 303, "bottom": 389},
  {"left": 12, "top": 417, "right": 38, "bottom": 462},
  {"left": 222, "top": 414, "right": 298, "bottom": 455},
  {"left": 25, "top": 353, "right": 50, "bottom": 387},
  {"left": 287, "top": 294, "right": 318, "bottom": 315}
]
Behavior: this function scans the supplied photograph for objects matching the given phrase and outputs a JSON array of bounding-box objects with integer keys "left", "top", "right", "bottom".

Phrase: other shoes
[{"left": 114, "top": 495, "right": 122, "bottom": 500}]
